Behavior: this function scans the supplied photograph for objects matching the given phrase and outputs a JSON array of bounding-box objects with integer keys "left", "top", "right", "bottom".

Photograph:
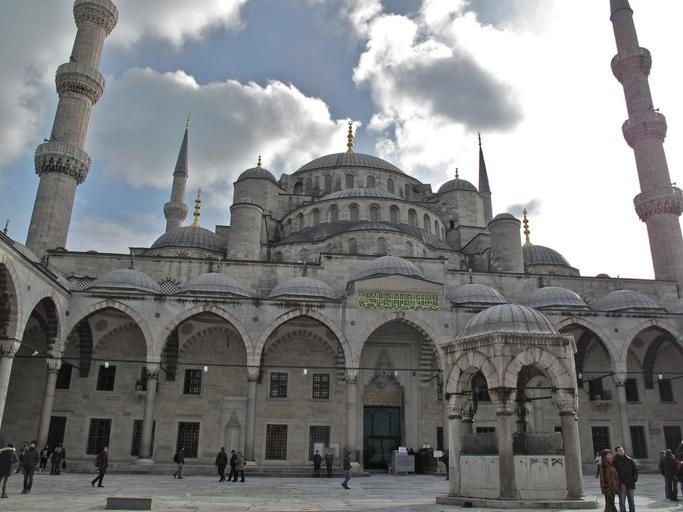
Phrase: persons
[
  {"left": 341, "top": 451, "right": 352, "bottom": 489},
  {"left": 173, "top": 447, "right": 184, "bottom": 479},
  {"left": 314, "top": 449, "right": 321, "bottom": 478},
  {"left": 595, "top": 445, "right": 683, "bottom": 512},
  {"left": 90, "top": 445, "right": 108, "bottom": 487},
  {"left": 214, "top": 447, "right": 227, "bottom": 482},
  {"left": 227, "top": 450, "right": 237, "bottom": 481},
  {"left": 325, "top": 450, "right": 334, "bottom": 477},
  {"left": 442, "top": 450, "right": 449, "bottom": 481},
  {"left": 231, "top": 451, "right": 247, "bottom": 482},
  {"left": 0, "top": 438, "right": 66, "bottom": 498}
]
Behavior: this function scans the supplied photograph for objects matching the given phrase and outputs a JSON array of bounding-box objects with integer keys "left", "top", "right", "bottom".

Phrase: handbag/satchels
[{"left": 61, "top": 460, "right": 66, "bottom": 469}]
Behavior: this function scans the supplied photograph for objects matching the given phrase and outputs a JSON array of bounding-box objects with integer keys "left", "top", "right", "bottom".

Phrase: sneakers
[
  {"left": 0, "top": 469, "right": 61, "bottom": 498},
  {"left": 312, "top": 474, "right": 350, "bottom": 489},
  {"left": 90, "top": 481, "right": 104, "bottom": 487},
  {"left": 172, "top": 473, "right": 245, "bottom": 482}
]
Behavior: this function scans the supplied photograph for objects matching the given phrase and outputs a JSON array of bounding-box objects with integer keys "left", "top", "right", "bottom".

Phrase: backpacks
[
  {"left": 173, "top": 453, "right": 179, "bottom": 463},
  {"left": 0, "top": 454, "right": 10, "bottom": 469},
  {"left": 93, "top": 454, "right": 100, "bottom": 466}
]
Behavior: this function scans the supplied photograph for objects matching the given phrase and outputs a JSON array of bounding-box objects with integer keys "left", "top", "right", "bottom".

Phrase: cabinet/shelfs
[{"left": 391, "top": 450, "right": 415, "bottom": 476}]
